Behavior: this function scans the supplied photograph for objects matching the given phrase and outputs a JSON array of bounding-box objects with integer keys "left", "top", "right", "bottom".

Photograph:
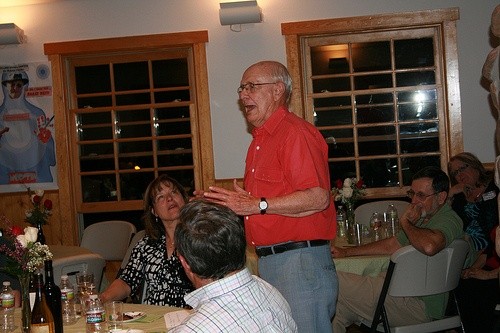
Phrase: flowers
[
  {"left": 0, "top": 216, "right": 53, "bottom": 327},
  {"left": 24, "top": 190, "right": 53, "bottom": 224},
  {"left": 331, "top": 177, "right": 368, "bottom": 204}
]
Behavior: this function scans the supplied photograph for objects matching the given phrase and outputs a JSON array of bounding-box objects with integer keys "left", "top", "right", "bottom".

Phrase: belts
[{"left": 256, "top": 238, "right": 330, "bottom": 257}]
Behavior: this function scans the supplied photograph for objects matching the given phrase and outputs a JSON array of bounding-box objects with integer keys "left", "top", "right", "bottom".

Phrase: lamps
[
  {"left": 0, "top": 23, "right": 24, "bottom": 45},
  {"left": 219, "top": 0, "right": 262, "bottom": 26},
  {"left": 328, "top": 58, "right": 347, "bottom": 74}
]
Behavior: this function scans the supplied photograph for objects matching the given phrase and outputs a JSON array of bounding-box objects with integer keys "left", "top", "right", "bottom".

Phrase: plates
[
  {"left": 106, "top": 312, "right": 145, "bottom": 323},
  {"left": 107, "top": 328, "right": 144, "bottom": 333}
]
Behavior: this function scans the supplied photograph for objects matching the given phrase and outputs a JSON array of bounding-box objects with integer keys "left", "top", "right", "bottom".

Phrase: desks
[
  {"left": 0, "top": 304, "right": 190, "bottom": 333},
  {"left": 48, "top": 244, "right": 108, "bottom": 293},
  {"left": 334, "top": 254, "right": 390, "bottom": 332}
]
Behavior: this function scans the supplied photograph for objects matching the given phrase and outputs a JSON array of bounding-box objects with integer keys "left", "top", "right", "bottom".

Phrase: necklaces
[{"left": 167, "top": 242, "right": 173, "bottom": 249}]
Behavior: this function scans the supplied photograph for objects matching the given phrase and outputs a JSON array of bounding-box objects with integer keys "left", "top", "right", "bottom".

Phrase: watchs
[{"left": 258, "top": 198, "right": 268, "bottom": 215}]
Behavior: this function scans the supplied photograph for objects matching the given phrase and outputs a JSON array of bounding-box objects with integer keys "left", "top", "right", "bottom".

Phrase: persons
[
  {"left": 446, "top": 152, "right": 500, "bottom": 333},
  {"left": 1, "top": 74, "right": 28, "bottom": 99},
  {"left": 189, "top": 61, "right": 339, "bottom": 333},
  {"left": 331, "top": 166, "right": 464, "bottom": 333},
  {"left": 0, "top": 271, "right": 21, "bottom": 307},
  {"left": 100, "top": 175, "right": 197, "bottom": 309},
  {"left": 167, "top": 200, "right": 299, "bottom": 333}
]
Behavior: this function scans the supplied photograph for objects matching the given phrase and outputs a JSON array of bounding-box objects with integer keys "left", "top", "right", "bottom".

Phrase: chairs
[
  {"left": 52, "top": 254, "right": 106, "bottom": 293},
  {"left": 354, "top": 201, "right": 410, "bottom": 225},
  {"left": 369, "top": 239, "right": 469, "bottom": 333},
  {"left": 81, "top": 221, "right": 137, "bottom": 260}
]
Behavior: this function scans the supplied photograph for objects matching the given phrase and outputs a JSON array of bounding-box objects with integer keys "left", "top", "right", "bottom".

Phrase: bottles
[
  {"left": 370, "top": 203, "right": 397, "bottom": 242},
  {"left": 0, "top": 282, "right": 15, "bottom": 333},
  {"left": 84, "top": 283, "right": 106, "bottom": 333},
  {"left": 59, "top": 275, "right": 76, "bottom": 325},
  {"left": 336, "top": 206, "right": 347, "bottom": 239},
  {"left": 25, "top": 260, "right": 63, "bottom": 333}
]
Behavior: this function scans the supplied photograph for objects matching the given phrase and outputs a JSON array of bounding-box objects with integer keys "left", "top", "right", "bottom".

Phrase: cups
[
  {"left": 353, "top": 224, "right": 363, "bottom": 245},
  {"left": 75, "top": 272, "right": 97, "bottom": 319},
  {"left": 389, "top": 218, "right": 400, "bottom": 236},
  {"left": 103, "top": 301, "right": 123, "bottom": 333}
]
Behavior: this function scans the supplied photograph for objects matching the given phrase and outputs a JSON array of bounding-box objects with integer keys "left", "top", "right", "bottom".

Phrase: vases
[
  {"left": 19, "top": 275, "right": 31, "bottom": 333},
  {"left": 37, "top": 223, "right": 45, "bottom": 245}
]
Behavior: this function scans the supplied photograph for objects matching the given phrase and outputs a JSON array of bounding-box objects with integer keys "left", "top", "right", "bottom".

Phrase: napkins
[{"left": 134, "top": 314, "right": 164, "bottom": 323}]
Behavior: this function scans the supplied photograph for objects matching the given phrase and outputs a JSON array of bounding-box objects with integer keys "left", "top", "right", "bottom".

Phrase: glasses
[
  {"left": 452, "top": 163, "right": 471, "bottom": 177},
  {"left": 238, "top": 82, "right": 276, "bottom": 94},
  {"left": 407, "top": 189, "right": 443, "bottom": 201}
]
